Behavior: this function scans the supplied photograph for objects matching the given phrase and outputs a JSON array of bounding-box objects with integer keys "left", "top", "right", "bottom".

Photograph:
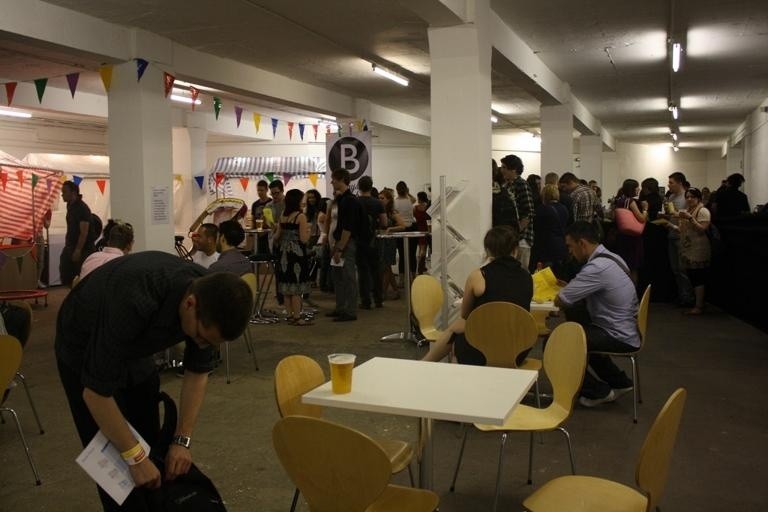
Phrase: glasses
[
  {"left": 191, "top": 313, "right": 220, "bottom": 357},
  {"left": 269, "top": 190, "right": 283, "bottom": 197}
]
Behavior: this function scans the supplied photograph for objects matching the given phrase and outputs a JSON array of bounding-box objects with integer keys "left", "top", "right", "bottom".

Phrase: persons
[
  {"left": 59, "top": 181, "right": 97, "bottom": 288},
  {"left": 392, "top": 182, "right": 417, "bottom": 272},
  {"left": 489, "top": 159, "right": 519, "bottom": 260},
  {"left": 638, "top": 177, "right": 666, "bottom": 279},
  {"left": 500, "top": 155, "right": 534, "bottom": 269},
  {"left": 254, "top": 181, "right": 272, "bottom": 227},
  {"left": 415, "top": 227, "right": 534, "bottom": 368},
  {"left": 57, "top": 252, "right": 252, "bottom": 511},
  {"left": 524, "top": 174, "right": 540, "bottom": 207},
  {"left": 174, "top": 222, "right": 255, "bottom": 377},
  {"left": 306, "top": 189, "right": 328, "bottom": 254},
  {"left": 264, "top": 182, "right": 284, "bottom": 249},
  {"left": 326, "top": 171, "right": 360, "bottom": 321},
  {"left": 35, "top": 206, "right": 52, "bottom": 287},
  {"left": 652, "top": 173, "right": 694, "bottom": 254},
  {"left": 669, "top": 188, "right": 712, "bottom": 314},
  {"left": 543, "top": 172, "right": 558, "bottom": 186},
  {"left": 554, "top": 221, "right": 639, "bottom": 406},
  {"left": 280, "top": 191, "right": 314, "bottom": 326},
  {"left": 709, "top": 174, "right": 750, "bottom": 280},
  {"left": 71, "top": 224, "right": 135, "bottom": 289},
  {"left": 700, "top": 187, "right": 714, "bottom": 218},
  {"left": 376, "top": 190, "right": 406, "bottom": 302},
  {"left": 561, "top": 172, "right": 602, "bottom": 225},
  {"left": 611, "top": 180, "right": 647, "bottom": 293},
  {"left": 355, "top": 175, "right": 388, "bottom": 310},
  {"left": 411, "top": 192, "right": 431, "bottom": 272},
  {"left": 186, "top": 225, "right": 219, "bottom": 267},
  {"left": 535, "top": 186, "right": 569, "bottom": 274}
]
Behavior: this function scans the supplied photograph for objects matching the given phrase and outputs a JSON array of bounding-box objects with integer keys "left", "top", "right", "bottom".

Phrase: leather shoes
[
  {"left": 324, "top": 311, "right": 337, "bottom": 318},
  {"left": 376, "top": 300, "right": 383, "bottom": 309},
  {"left": 331, "top": 317, "right": 345, "bottom": 323},
  {"left": 577, "top": 389, "right": 617, "bottom": 408},
  {"left": 302, "top": 311, "right": 313, "bottom": 316},
  {"left": 358, "top": 302, "right": 371, "bottom": 311}
]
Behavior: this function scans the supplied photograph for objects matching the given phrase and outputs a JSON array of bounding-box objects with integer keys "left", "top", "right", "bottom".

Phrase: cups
[
  {"left": 256, "top": 219, "right": 263, "bottom": 231},
  {"left": 328, "top": 353, "right": 356, "bottom": 394}
]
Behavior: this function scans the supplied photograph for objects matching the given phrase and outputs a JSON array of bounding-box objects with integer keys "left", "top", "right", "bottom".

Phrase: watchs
[{"left": 168, "top": 436, "right": 193, "bottom": 448}]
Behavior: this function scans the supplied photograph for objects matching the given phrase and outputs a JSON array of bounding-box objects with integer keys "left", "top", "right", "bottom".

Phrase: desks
[{"left": 376, "top": 233, "right": 436, "bottom": 342}]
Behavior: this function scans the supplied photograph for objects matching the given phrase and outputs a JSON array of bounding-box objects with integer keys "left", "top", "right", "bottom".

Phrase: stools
[
  {"left": 248, "top": 253, "right": 280, "bottom": 313},
  {"left": 296, "top": 259, "right": 322, "bottom": 325}
]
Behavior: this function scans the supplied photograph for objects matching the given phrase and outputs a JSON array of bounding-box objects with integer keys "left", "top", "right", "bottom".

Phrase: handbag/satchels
[{"left": 612, "top": 196, "right": 648, "bottom": 236}]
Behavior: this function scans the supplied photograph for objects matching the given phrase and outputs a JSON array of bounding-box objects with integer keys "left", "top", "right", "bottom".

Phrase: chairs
[
  {"left": 0, "top": 334, "right": 41, "bottom": 489},
  {"left": 590, "top": 283, "right": 652, "bottom": 422},
  {"left": 224, "top": 273, "right": 258, "bottom": 385},
  {"left": 463, "top": 302, "right": 541, "bottom": 409},
  {"left": 521, "top": 386, "right": 688, "bottom": 512},
  {"left": 448, "top": 323, "right": 586, "bottom": 507},
  {"left": 1, "top": 299, "right": 45, "bottom": 434},
  {"left": 274, "top": 352, "right": 414, "bottom": 512},
  {"left": 263, "top": 416, "right": 442, "bottom": 512},
  {"left": 411, "top": 275, "right": 455, "bottom": 344}
]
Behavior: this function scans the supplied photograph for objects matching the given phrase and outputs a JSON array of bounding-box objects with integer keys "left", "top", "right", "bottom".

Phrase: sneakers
[
  {"left": 609, "top": 370, "right": 634, "bottom": 399},
  {"left": 303, "top": 299, "right": 318, "bottom": 308},
  {"left": 692, "top": 307, "right": 702, "bottom": 313}
]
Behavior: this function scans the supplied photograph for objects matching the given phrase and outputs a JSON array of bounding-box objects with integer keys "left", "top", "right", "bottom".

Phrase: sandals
[
  {"left": 294, "top": 317, "right": 314, "bottom": 328},
  {"left": 286, "top": 316, "right": 296, "bottom": 326}
]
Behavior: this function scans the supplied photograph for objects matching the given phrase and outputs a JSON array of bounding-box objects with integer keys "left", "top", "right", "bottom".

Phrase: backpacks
[
  {"left": 339, "top": 192, "right": 376, "bottom": 254},
  {"left": 84, "top": 393, "right": 225, "bottom": 511}
]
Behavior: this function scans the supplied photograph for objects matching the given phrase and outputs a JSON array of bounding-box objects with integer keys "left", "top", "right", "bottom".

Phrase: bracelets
[{"left": 119, "top": 442, "right": 147, "bottom": 466}]
